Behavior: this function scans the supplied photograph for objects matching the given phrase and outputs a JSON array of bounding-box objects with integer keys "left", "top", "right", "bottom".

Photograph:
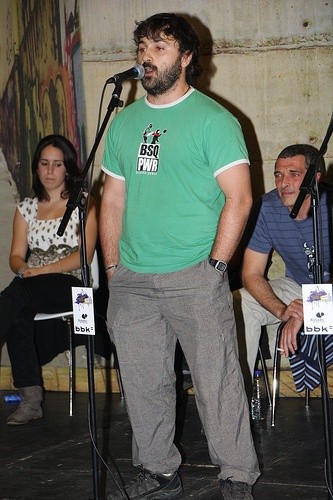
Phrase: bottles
[{"left": 251, "top": 370, "right": 268, "bottom": 421}]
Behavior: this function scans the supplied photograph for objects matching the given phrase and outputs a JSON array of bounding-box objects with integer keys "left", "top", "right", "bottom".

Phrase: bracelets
[
  {"left": 17, "top": 268, "right": 25, "bottom": 279},
  {"left": 58, "top": 259, "right": 65, "bottom": 274},
  {"left": 106, "top": 264, "right": 118, "bottom": 271}
]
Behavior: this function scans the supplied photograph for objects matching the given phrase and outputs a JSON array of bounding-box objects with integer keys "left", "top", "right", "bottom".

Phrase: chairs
[{"left": 62, "top": 241, "right": 123, "bottom": 415}]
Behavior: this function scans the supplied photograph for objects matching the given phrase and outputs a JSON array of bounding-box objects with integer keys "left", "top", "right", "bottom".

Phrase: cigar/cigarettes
[{"left": 277, "top": 348, "right": 285, "bottom": 352}]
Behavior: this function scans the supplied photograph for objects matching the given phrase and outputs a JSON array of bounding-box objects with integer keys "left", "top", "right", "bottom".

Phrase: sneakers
[
  {"left": 106, "top": 464, "right": 182, "bottom": 500},
  {"left": 220, "top": 476, "right": 253, "bottom": 500}
]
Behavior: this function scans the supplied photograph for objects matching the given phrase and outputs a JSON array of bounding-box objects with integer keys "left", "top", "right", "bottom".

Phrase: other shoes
[{"left": 186, "top": 418, "right": 204, "bottom": 439}]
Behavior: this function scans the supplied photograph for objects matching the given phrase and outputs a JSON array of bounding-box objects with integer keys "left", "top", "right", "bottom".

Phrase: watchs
[{"left": 209, "top": 258, "right": 228, "bottom": 275}]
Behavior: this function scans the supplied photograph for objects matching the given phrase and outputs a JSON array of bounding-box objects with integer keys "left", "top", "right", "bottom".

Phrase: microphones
[{"left": 106, "top": 64, "right": 145, "bottom": 85}]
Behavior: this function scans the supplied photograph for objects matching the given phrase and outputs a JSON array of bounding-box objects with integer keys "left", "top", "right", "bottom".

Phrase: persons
[
  {"left": 231, "top": 144, "right": 333, "bottom": 397},
  {"left": 0, "top": 133, "right": 100, "bottom": 426},
  {"left": 97, "top": 13, "right": 262, "bottom": 500}
]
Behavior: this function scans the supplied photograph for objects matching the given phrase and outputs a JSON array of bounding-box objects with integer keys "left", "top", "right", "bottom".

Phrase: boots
[{"left": 6, "top": 385, "right": 42, "bottom": 424}]
